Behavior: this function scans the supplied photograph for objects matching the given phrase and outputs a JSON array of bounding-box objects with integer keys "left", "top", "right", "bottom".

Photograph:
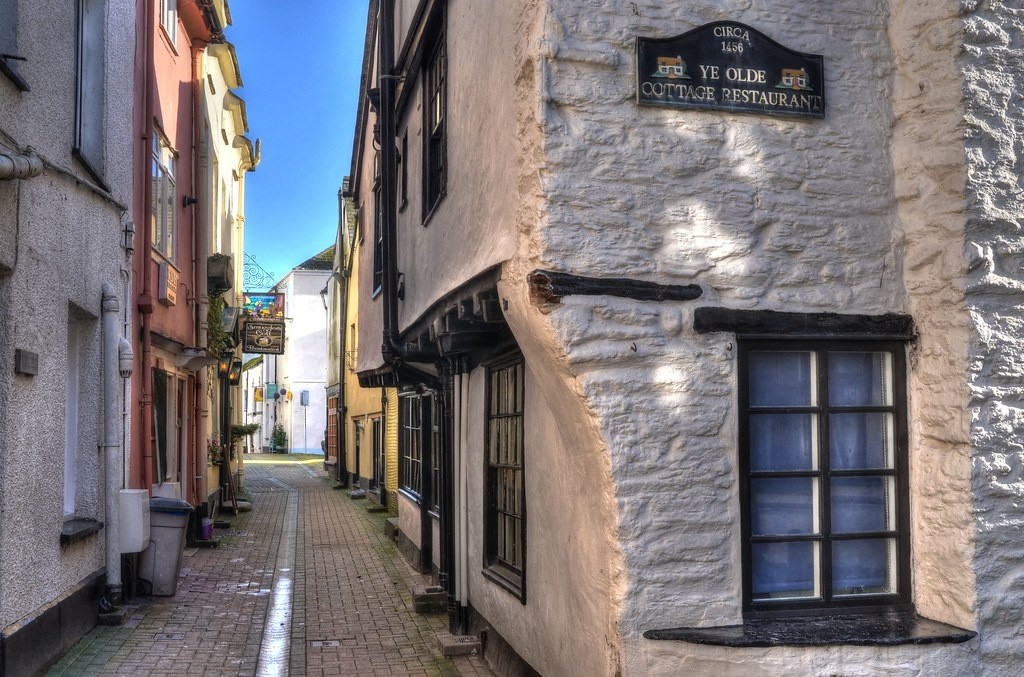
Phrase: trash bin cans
[
  {"left": 203, "top": 519, "right": 214, "bottom": 541},
  {"left": 134, "top": 495, "right": 195, "bottom": 600}
]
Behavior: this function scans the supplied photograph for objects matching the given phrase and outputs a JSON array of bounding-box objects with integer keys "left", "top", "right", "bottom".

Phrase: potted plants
[
  {"left": 274, "top": 421, "right": 286, "bottom": 454},
  {"left": 208, "top": 250, "right": 234, "bottom": 289}
]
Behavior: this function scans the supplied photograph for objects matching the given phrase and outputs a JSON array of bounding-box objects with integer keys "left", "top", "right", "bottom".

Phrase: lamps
[{"left": 216, "top": 345, "right": 243, "bottom": 386}]
